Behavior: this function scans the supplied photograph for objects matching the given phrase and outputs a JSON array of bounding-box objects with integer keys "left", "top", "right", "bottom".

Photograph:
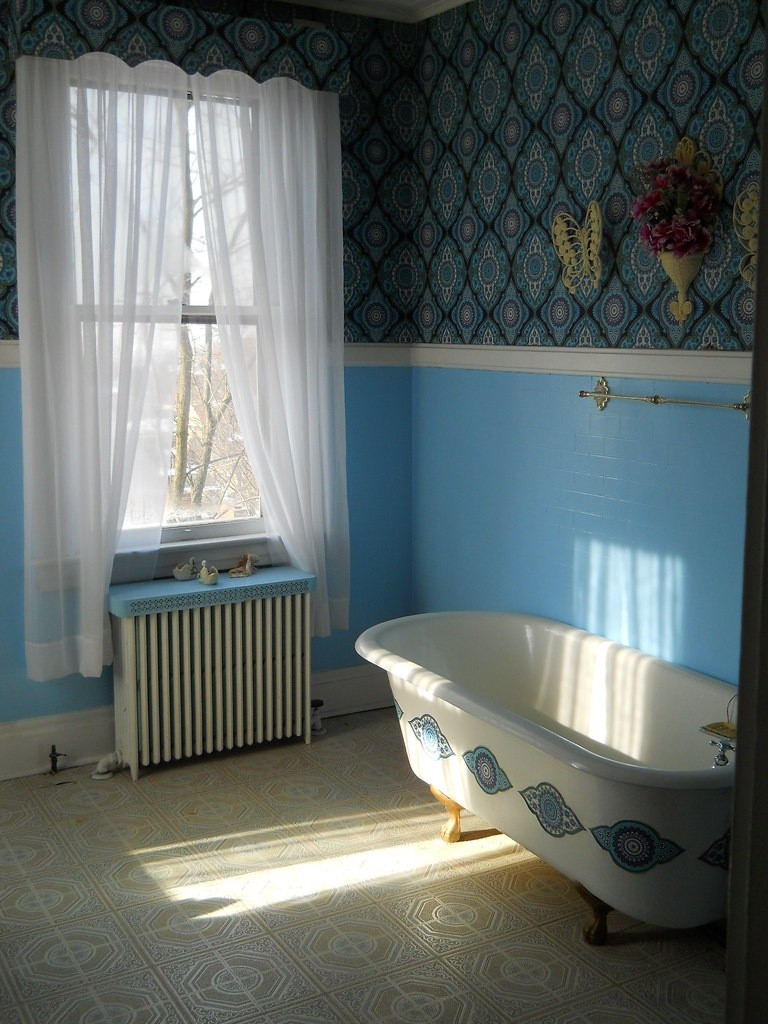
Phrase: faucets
[{"left": 709, "top": 739, "right": 735, "bottom": 768}]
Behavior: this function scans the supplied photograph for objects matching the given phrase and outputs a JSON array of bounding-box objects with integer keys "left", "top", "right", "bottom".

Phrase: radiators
[{"left": 110, "top": 592, "right": 311, "bottom": 782}]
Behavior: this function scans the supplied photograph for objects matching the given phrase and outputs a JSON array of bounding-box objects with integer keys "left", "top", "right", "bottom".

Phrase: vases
[{"left": 661, "top": 251, "right": 706, "bottom": 321}]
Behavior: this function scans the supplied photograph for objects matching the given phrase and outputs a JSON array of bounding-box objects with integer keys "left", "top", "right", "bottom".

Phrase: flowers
[{"left": 630, "top": 154, "right": 719, "bottom": 260}]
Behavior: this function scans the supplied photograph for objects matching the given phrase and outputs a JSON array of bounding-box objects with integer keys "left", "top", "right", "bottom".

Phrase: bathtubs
[{"left": 354, "top": 610, "right": 740, "bottom": 946}]
